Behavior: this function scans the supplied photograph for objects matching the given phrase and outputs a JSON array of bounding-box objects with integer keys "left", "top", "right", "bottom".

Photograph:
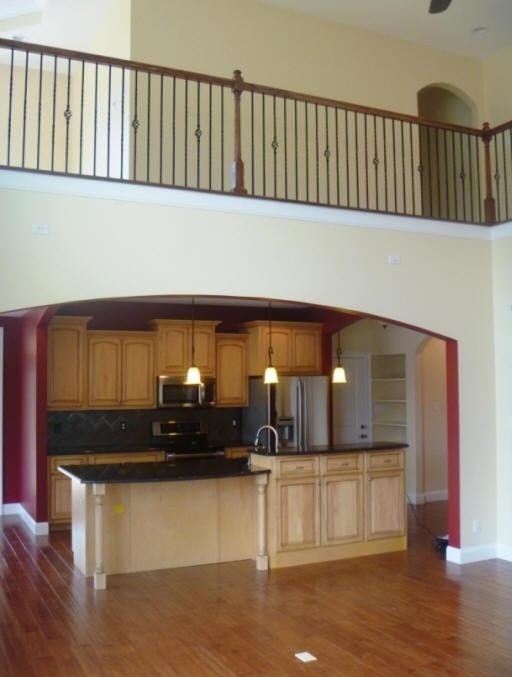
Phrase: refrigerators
[{"left": 241, "top": 375, "right": 330, "bottom": 448}]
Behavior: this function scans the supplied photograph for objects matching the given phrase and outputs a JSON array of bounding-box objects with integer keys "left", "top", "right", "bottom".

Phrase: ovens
[{"left": 165, "top": 450, "right": 224, "bottom": 462}]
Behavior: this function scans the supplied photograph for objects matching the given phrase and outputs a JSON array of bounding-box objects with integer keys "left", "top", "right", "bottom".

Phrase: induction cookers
[{"left": 155, "top": 442, "right": 223, "bottom": 450}]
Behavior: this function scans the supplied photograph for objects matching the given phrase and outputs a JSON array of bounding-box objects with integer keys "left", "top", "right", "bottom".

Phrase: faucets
[{"left": 253, "top": 425, "right": 279, "bottom": 454}]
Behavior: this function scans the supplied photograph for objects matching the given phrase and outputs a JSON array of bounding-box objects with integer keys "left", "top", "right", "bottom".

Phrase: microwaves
[{"left": 158, "top": 376, "right": 216, "bottom": 409}]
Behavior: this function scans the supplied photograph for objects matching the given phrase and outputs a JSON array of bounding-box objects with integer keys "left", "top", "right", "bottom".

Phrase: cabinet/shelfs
[
  {"left": 370, "top": 350, "right": 408, "bottom": 443},
  {"left": 88, "top": 325, "right": 215, "bottom": 409},
  {"left": 215, "top": 336, "right": 250, "bottom": 407},
  {"left": 271, "top": 447, "right": 408, "bottom": 569},
  {"left": 263, "top": 325, "right": 322, "bottom": 375},
  {"left": 49, "top": 454, "right": 89, "bottom": 531},
  {"left": 89, "top": 450, "right": 165, "bottom": 465},
  {"left": 43, "top": 322, "right": 88, "bottom": 412}
]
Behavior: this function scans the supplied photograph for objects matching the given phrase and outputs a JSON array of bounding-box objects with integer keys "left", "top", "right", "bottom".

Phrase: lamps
[{"left": 184, "top": 295, "right": 367, "bottom": 387}]
